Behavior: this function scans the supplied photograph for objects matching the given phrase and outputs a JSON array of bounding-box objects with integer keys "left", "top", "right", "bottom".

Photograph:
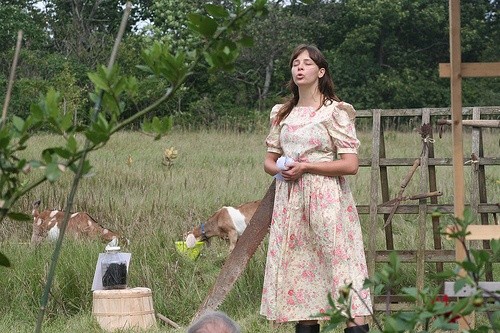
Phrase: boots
[
  {"left": 296, "top": 323, "right": 320, "bottom": 333},
  {"left": 344, "top": 323, "right": 371, "bottom": 333}
]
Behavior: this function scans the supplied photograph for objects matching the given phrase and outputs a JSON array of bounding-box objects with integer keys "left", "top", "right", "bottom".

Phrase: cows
[
  {"left": 30, "top": 200, "right": 123, "bottom": 244},
  {"left": 186, "top": 200, "right": 267, "bottom": 257}
]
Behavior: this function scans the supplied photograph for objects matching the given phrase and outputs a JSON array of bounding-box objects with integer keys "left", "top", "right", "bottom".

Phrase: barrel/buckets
[
  {"left": 92, "top": 283, "right": 157, "bottom": 331},
  {"left": 175, "top": 238, "right": 205, "bottom": 264}
]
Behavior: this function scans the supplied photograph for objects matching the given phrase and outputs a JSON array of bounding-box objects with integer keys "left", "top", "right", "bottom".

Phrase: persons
[{"left": 259, "top": 42, "right": 375, "bottom": 333}]
